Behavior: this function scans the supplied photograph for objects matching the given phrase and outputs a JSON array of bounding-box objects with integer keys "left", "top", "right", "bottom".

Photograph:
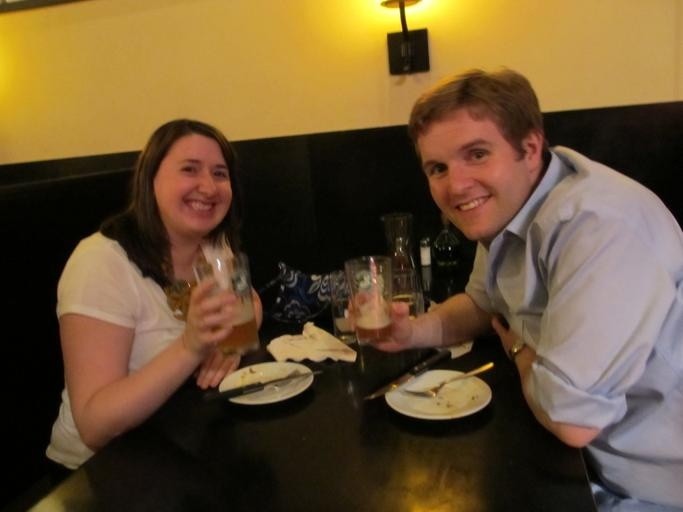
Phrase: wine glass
[{"left": 432, "top": 211, "right": 461, "bottom": 267}]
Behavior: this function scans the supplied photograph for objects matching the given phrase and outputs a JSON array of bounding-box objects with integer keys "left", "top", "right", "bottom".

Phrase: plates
[
  {"left": 385, "top": 369, "right": 493, "bottom": 420},
  {"left": 216, "top": 361, "right": 314, "bottom": 405}
]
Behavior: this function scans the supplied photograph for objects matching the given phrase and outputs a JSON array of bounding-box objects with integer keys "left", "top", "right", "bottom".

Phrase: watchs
[{"left": 509, "top": 337, "right": 526, "bottom": 358}]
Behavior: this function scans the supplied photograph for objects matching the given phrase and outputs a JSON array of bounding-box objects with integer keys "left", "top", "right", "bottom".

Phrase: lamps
[{"left": 381, "top": 0, "right": 430, "bottom": 76}]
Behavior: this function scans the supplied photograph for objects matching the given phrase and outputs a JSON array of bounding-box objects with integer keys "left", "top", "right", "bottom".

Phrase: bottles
[
  {"left": 379, "top": 211, "right": 421, "bottom": 319},
  {"left": 419, "top": 235, "right": 433, "bottom": 268}
]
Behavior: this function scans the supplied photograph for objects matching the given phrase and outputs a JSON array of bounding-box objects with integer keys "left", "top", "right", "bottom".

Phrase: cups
[
  {"left": 344, "top": 254, "right": 393, "bottom": 346},
  {"left": 328, "top": 268, "right": 359, "bottom": 347},
  {"left": 190, "top": 249, "right": 259, "bottom": 355}
]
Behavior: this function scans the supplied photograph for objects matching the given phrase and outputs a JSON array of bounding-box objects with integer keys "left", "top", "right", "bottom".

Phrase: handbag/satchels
[{"left": 258, "top": 262, "right": 345, "bottom": 323}]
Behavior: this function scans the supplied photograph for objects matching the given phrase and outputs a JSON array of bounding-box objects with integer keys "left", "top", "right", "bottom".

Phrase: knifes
[
  {"left": 203, "top": 370, "right": 323, "bottom": 402},
  {"left": 363, "top": 349, "right": 451, "bottom": 401}
]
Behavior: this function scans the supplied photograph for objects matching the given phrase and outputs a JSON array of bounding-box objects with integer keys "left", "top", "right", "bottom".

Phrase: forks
[{"left": 405, "top": 362, "right": 494, "bottom": 398}]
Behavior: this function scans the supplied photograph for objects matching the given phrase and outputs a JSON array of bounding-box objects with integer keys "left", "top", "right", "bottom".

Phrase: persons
[
  {"left": 347, "top": 67, "right": 682, "bottom": 511},
  {"left": 42, "top": 120, "right": 263, "bottom": 493}
]
